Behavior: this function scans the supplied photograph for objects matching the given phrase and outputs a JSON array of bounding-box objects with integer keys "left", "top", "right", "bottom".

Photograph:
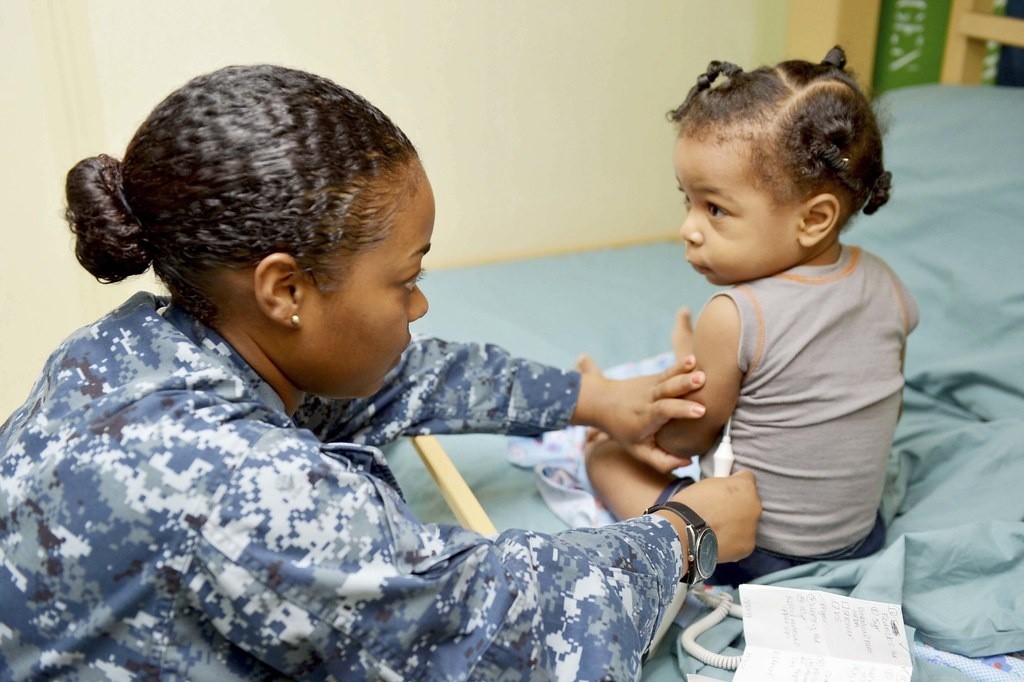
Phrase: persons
[
  {"left": 0, "top": 62, "right": 764, "bottom": 682},
  {"left": 580, "top": 43, "right": 921, "bottom": 590}
]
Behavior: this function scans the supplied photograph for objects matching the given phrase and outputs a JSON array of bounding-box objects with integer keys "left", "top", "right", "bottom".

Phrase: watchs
[{"left": 644, "top": 501, "right": 719, "bottom": 586}]
